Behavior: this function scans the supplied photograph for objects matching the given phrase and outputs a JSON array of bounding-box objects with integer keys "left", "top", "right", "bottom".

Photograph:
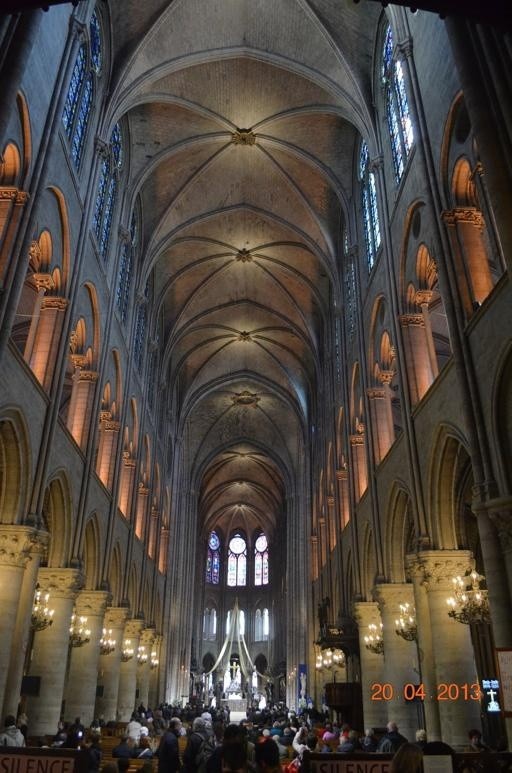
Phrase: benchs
[
  {"left": 0, "top": 716, "right": 188, "bottom": 773},
  {"left": 242, "top": 723, "right": 483, "bottom": 773}
]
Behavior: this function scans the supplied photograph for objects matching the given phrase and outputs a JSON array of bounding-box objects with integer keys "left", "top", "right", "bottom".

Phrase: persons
[{"left": 462, "top": 728, "right": 494, "bottom": 752}]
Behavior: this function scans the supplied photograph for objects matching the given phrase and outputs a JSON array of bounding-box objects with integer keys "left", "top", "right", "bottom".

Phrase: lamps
[
  {"left": 315, "top": 568, "right": 489, "bottom": 669},
  {"left": 30, "top": 589, "right": 160, "bottom": 669}
]
